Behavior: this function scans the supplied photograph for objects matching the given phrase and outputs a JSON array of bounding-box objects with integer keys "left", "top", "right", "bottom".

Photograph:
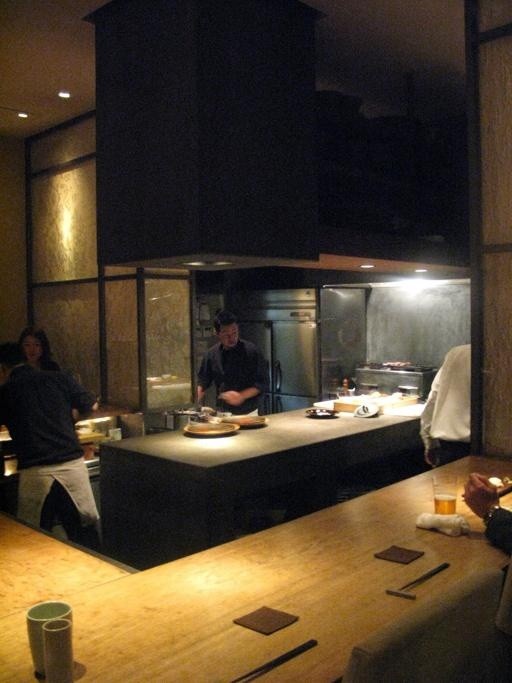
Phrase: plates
[
  {"left": 306, "top": 409, "right": 340, "bottom": 417},
  {"left": 145, "top": 373, "right": 179, "bottom": 384},
  {"left": 183, "top": 423, "right": 241, "bottom": 436},
  {"left": 219, "top": 415, "right": 271, "bottom": 427}
]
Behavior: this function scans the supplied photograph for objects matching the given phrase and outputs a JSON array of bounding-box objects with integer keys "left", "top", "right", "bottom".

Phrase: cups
[
  {"left": 359, "top": 382, "right": 377, "bottom": 395},
  {"left": 5, "top": 455, "right": 19, "bottom": 476},
  {"left": 109, "top": 427, "right": 122, "bottom": 441},
  {"left": 399, "top": 385, "right": 420, "bottom": 398},
  {"left": 80, "top": 443, "right": 94, "bottom": 459},
  {"left": 41, "top": 618, "right": 74, "bottom": 683},
  {"left": 430, "top": 470, "right": 458, "bottom": 519},
  {"left": 335, "top": 387, "right": 355, "bottom": 398},
  {"left": 25, "top": 599, "right": 74, "bottom": 674}
]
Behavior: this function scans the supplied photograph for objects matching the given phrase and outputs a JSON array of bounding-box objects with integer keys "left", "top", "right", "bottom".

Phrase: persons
[
  {"left": 1, "top": 339, "right": 103, "bottom": 548},
  {"left": 418, "top": 344, "right": 470, "bottom": 470},
  {"left": 193, "top": 307, "right": 262, "bottom": 416},
  {"left": 19, "top": 324, "right": 73, "bottom": 538}
]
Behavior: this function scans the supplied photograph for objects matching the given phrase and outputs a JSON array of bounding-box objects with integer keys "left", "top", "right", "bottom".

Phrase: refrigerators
[{"left": 235, "top": 284, "right": 369, "bottom": 416}]
